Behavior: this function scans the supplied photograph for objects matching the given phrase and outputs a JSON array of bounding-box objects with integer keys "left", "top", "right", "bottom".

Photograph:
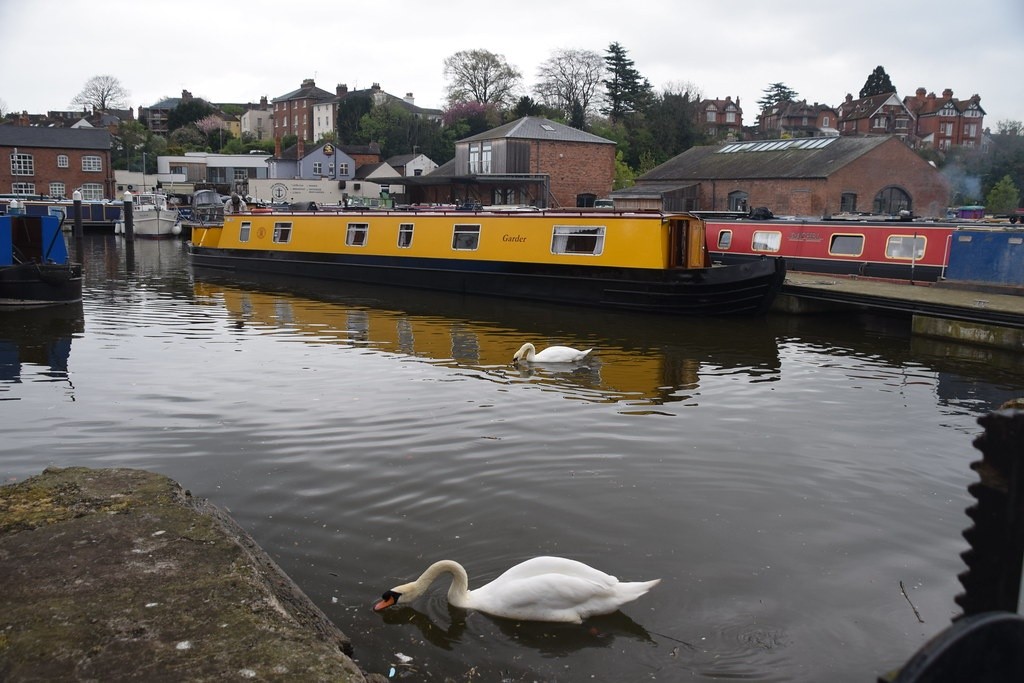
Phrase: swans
[
  {"left": 513, "top": 343, "right": 594, "bottom": 364},
  {"left": 375, "top": 556, "right": 662, "bottom": 624}
]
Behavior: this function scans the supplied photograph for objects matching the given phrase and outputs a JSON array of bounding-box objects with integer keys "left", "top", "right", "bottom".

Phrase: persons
[{"left": 222, "top": 194, "right": 248, "bottom": 215}]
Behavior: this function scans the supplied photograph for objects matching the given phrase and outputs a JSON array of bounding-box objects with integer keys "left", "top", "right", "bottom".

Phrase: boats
[
  {"left": 192, "top": 199, "right": 791, "bottom": 319},
  {"left": 0, "top": 199, "right": 85, "bottom": 307},
  {"left": 687, "top": 208, "right": 1023, "bottom": 325},
  {"left": 115, "top": 193, "right": 182, "bottom": 236}
]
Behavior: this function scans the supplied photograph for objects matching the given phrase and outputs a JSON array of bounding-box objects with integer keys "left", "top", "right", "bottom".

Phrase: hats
[{"left": 232, "top": 194, "right": 240, "bottom": 205}]
